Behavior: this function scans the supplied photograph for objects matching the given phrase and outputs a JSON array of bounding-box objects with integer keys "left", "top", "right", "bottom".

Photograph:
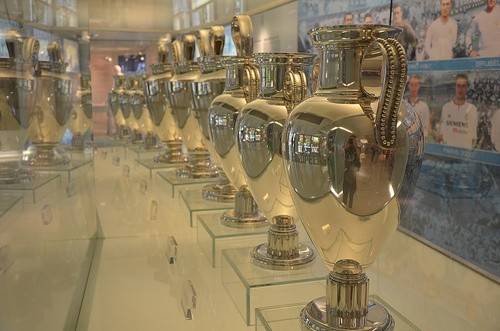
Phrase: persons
[
  {"left": 404, "top": 73, "right": 430, "bottom": 136},
  {"left": 391, "top": 3, "right": 418, "bottom": 60},
  {"left": 341, "top": 134, "right": 361, "bottom": 208},
  {"left": 362, "top": 13, "right": 373, "bottom": 22},
  {"left": 342, "top": 11, "right": 353, "bottom": 24},
  {"left": 440, "top": 73, "right": 479, "bottom": 150},
  {"left": 463, "top": 0, "right": 499, "bottom": 58},
  {"left": 424, "top": 0, "right": 459, "bottom": 60}
]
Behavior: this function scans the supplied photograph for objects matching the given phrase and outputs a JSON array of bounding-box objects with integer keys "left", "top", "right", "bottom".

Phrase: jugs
[
  {"left": 1, "top": 37, "right": 93, "bottom": 187},
  {"left": 107, "top": 16, "right": 425, "bottom": 331}
]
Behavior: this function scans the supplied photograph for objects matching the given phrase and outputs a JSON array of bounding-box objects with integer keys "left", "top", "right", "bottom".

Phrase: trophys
[
  {"left": 0, "top": 33, "right": 94, "bottom": 172},
  {"left": 234, "top": 50, "right": 317, "bottom": 271},
  {"left": 105, "top": 15, "right": 256, "bottom": 205},
  {"left": 282, "top": 23, "right": 423, "bottom": 331}
]
